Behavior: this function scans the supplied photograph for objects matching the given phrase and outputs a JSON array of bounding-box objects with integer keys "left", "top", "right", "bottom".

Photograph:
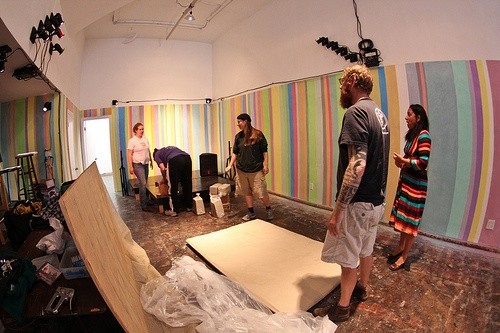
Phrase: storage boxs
[{"left": 59, "top": 240, "right": 90, "bottom": 279}]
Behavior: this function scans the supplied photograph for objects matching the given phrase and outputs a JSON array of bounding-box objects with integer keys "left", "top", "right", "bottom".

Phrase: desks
[{"left": 130, "top": 169, "right": 235, "bottom": 214}]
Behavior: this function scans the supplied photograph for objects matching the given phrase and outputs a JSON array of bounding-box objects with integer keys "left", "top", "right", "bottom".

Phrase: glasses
[{"left": 338, "top": 77, "right": 346, "bottom": 85}]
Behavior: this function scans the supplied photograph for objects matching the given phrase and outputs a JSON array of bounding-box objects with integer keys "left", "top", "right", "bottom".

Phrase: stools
[
  {"left": 15, "top": 154, "right": 39, "bottom": 201},
  {"left": 0, "top": 165, "right": 27, "bottom": 205}
]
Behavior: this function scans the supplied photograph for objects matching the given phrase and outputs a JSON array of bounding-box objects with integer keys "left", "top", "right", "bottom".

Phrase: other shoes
[
  {"left": 314, "top": 302, "right": 350, "bottom": 322},
  {"left": 267, "top": 209, "right": 274, "bottom": 220},
  {"left": 354, "top": 281, "right": 368, "bottom": 300},
  {"left": 142, "top": 207, "right": 150, "bottom": 212},
  {"left": 164, "top": 210, "right": 177, "bottom": 216},
  {"left": 187, "top": 208, "right": 192, "bottom": 211},
  {"left": 242, "top": 211, "right": 256, "bottom": 221}
]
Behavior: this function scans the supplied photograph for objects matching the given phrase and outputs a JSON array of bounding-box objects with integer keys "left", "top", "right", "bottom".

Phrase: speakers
[{"left": 199, "top": 153, "right": 218, "bottom": 177}]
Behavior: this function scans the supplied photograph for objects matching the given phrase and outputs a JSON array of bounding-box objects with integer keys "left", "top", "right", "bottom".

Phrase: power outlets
[{"left": 309, "top": 183, "right": 313, "bottom": 190}]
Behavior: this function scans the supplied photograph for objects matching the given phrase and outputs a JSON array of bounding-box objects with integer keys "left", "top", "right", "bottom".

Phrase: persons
[
  {"left": 127, "top": 123, "right": 153, "bottom": 211},
  {"left": 386, "top": 103, "right": 431, "bottom": 270},
  {"left": 312, "top": 64, "right": 390, "bottom": 322},
  {"left": 223, "top": 114, "right": 273, "bottom": 222},
  {"left": 153, "top": 146, "right": 193, "bottom": 217}
]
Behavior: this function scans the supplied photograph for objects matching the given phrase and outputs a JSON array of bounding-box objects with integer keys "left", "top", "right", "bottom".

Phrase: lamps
[
  {"left": 30, "top": 12, "right": 65, "bottom": 81},
  {"left": 316, "top": 37, "right": 358, "bottom": 63},
  {"left": 42, "top": 102, "right": 51, "bottom": 112},
  {"left": 113, "top": 0, "right": 233, "bottom": 29},
  {"left": 206, "top": 98, "right": 211, "bottom": 103},
  {"left": 0, "top": 45, "right": 13, "bottom": 73},
  {"left": 112, "top": 100, "right": 118, "bottom": 106}
]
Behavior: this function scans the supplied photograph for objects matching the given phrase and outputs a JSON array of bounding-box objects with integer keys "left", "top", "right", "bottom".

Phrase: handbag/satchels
[{"left": 3, "top": 201, "right": 37, "bottom": 250}]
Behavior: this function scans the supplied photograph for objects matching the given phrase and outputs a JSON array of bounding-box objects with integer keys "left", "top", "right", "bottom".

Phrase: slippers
[
  {"left": 389, "top": 262, "right": 405, "bottom": 270},
  {"left": 388, "top": 250, "right": 405, "bottom": 259}
]
